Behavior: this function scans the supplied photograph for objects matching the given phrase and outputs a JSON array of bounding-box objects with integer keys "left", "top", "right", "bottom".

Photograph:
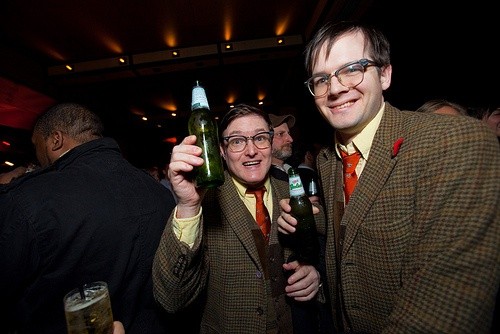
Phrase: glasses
[
  {"left": 221, "top": 131, "right": 274, "bottom": 152},
  {"left": 304, "top": 58, "right": 382, "bottom": 96}
]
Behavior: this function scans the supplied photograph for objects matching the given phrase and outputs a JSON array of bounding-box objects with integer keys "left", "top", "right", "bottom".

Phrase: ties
[
  {"left": 246, "top": 186, "right": 271, "bottom": 244},
  {"left": 337, "top": 147, "right": 361, "bottom": 205}
]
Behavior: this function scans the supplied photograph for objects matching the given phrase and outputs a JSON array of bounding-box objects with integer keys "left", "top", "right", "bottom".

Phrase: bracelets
[{"left": 316, "top": 270, "right": 323, "bottom": 289}]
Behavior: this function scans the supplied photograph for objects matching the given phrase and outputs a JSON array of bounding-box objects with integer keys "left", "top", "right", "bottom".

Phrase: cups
[{"left": 63, "top": 281, "right": 115, "bottom": 334}]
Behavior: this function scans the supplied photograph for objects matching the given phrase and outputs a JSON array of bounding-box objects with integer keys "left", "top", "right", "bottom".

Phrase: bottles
[
  {"left": 306, "top": 176, "right": 320, "bottom": 197},
  {"left": 288, "top": 165, "right": 322, "bottom": 260},
  {"left": 188, "top": 79, "right": 225, "bottom": 191}
]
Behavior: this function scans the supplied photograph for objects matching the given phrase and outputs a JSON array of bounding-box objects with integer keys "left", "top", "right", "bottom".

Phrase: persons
[
  {"left": 277, "top": 17, "right": 500, "bottom": 334},
  {"left": 295, "top": 139, "right": 325, "bottom": 202},
  {"left": 417, "top": 99, "right": 468, "bottom": 118},
  {"left": 478, "top": 102, "right": 500, "bottom": 137},
  {"left": 143, "top": 160, "right": 177, "bottom": 202},
  {"left": 267, "top": 114, "right": 296, "bottom": 176},
  {"left": 151, "top": 105, "right": 326, "bottom": 334},
  {"left": 0, "top": 103, "right": 176, "bottom": 334}
]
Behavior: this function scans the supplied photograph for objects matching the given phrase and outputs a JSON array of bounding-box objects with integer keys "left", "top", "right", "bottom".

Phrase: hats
[{"left": 268, "top": 113, "right": 294, "bottom": 129}]
